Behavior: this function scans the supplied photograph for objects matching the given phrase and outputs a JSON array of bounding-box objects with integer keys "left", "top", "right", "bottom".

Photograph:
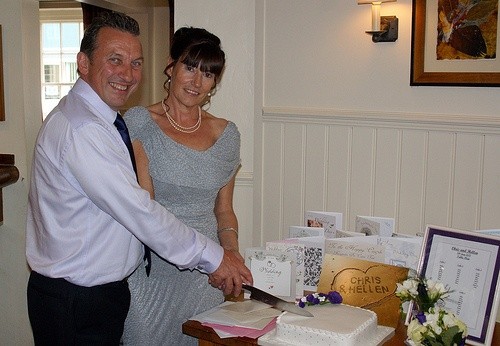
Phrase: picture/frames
[{"left": 410, "top": 0, "right": 500, "bottom": 87}]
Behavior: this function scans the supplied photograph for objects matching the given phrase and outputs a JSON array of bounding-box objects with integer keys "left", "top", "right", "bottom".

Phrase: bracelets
[{"left": 218, "top": 228, "right": 238, "bottom": 237}]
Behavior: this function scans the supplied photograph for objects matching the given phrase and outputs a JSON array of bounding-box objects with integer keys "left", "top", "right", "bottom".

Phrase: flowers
[
  {"left": 297, "top": 291, "right": 343, "bottom": 309},
  {"left": 395, "top": 277, "right": 470, "bottom": 346}
]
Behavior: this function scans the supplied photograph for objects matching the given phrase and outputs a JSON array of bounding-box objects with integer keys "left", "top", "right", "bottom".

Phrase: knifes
[{"left": 242, "top": 283, "right": 314, "bottom": 317}]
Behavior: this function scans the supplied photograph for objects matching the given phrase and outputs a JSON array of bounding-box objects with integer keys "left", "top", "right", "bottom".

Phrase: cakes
[{"left": 275, "top": 290, "right": 377, "bottom": 346}]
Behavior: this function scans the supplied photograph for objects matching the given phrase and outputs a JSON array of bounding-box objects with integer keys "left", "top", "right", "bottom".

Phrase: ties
[{"left": 113, "top": 114, "right": 153, "bottom": 277}]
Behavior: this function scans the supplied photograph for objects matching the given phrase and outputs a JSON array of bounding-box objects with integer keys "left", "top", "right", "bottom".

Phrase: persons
[
  {"left": 25, "top": 12, "right": 253, "bottom": 346},
  {"left": 120, "top": 25, "right": 245, "bottom": 346}
]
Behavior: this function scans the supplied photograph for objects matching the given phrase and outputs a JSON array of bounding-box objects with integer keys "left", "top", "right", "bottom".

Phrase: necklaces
[{"left": 162, "top": 99, "right": 201, "bottom": 133}]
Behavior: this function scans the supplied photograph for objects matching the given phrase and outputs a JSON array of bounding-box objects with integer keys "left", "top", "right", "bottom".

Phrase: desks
[{"left": 179, "top": 298, "right": 500, "bottom": 346}]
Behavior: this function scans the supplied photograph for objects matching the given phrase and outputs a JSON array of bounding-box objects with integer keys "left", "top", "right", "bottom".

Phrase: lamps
[{"left": 356, "top": 0, "right": 400, "bottom": 43}]
